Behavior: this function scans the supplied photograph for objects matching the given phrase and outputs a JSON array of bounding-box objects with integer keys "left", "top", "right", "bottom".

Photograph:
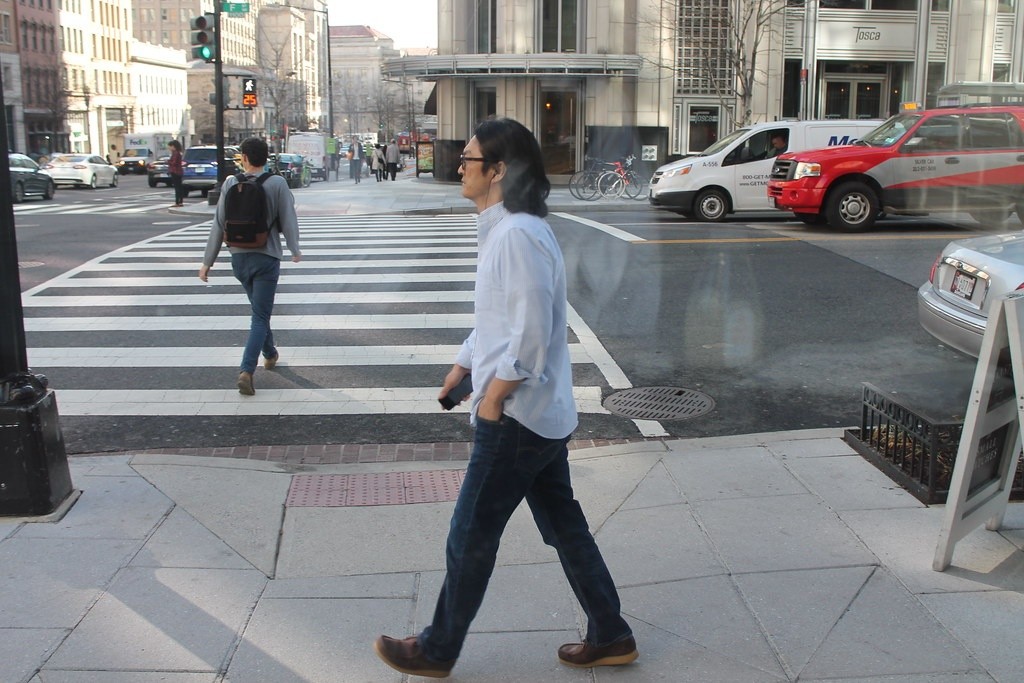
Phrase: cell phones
[{"left": 439, "top": 373, "right": 473, "bottom": 411}]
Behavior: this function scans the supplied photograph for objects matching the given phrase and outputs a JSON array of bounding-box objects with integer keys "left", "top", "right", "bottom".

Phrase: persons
[
  {"left": 370, "top": 138, "right": 401, "bottom": 182},
  {"left": 348, "top": 136, "right": 364, "bottom": 184},
  {"left": 199, "top": 137, "right": 302, "bottom": 396},
  {"left": 764, "top": 133, "right": 788, "bottom": 159},
  {"left": 374, "top": 118, "right": 639, "bottom": 679},
  {"left": 167, "top": 140, "right": 184, "bottom": 207},
  {"left": 20, "top": 152, "right": 51, "bottom": 166}
]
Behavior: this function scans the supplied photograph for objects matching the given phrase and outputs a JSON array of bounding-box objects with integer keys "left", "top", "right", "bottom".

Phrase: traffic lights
[
  {"left": 242, "top": 79, "right": 257, "bottom": 93},
  {"left": 191, "top": 16, "right": 203, "bottom": 59},
  {"left": 208, "top": 78, "right": 230, "bottom": 107},
  {"left": 195, "top": 14, "right": 215, "bottom": 60},
  {"left": 243, "top": 94, "right": 257, "bottom": 106}
]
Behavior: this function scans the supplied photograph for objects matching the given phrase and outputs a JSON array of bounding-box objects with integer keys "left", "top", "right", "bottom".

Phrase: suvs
[
  {"left": 766, "top": 102, "right": 1023, "bottom": 234},
  {"left": 180, "top": 144, "right": 244, "bottom": 198}
]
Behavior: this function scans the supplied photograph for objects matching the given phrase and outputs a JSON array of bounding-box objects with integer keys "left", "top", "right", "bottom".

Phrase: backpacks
[{"left": 223, "top": 173, "right": 277, "bottom": 248}]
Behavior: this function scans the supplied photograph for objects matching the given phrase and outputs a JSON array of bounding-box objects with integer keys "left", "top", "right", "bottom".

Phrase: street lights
[
  {"left": 380, "top": 78, "right": 416, "bottom": 143},
  {"left": 266, "top": 2, "right": 336, "bottom": 172}
]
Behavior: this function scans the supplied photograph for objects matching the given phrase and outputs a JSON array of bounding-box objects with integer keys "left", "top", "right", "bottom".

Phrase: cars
[
  {"left": 276, "top": 153, "right": 310, "bottom": 188},
  {"left": 233, "top": 145, "right": 277, "bottom": 175},
  {"left": 146, "top": 156, "right": 171, "bottom": 189},
  {"left": 916, "top": 229, "right": 1024, "bottom": 368},
  {"left": 42, "top": 153, "right": 119, "bottom": 189},
  {"left": 8, "top": 152, "right": 55, "bottom": 204},
  {"left": 338, "top": 145, "right": 373, "bottom": 158}
]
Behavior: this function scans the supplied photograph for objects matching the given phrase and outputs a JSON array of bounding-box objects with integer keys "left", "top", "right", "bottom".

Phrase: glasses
[{"left": 460, "top": 154, "right": 499, "bottom": 170}]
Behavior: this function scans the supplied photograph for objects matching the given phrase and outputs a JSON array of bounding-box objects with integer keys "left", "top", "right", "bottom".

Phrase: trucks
[
  {"left": 115, "top": 131, "right": 172, "bottom": 175},
  {"left": 362, "top": 132, "right": 378, "bottom": 147},
  {"left": 288, "top": 131, "right": 331, "bottom": 181}
]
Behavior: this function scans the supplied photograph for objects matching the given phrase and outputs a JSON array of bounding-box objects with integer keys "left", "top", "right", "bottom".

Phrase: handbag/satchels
[
  {"left": 348, "top": 152, "right": 353, "bottom": 159},
  {"left": 379, "top": 158, "right": 384, "bottom": 163}
]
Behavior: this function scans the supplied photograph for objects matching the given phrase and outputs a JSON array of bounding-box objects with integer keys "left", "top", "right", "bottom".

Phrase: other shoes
[
  {"left": 238, "top": 371, "right": 255, "bottom": 395},
  {"left": 264, "top": 352, "right": 278, "bottom": 370}
]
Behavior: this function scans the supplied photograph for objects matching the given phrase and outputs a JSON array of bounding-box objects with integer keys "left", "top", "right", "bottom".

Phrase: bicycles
[{"left": 568, "top": 154, "right": 651, "bottom": 201}]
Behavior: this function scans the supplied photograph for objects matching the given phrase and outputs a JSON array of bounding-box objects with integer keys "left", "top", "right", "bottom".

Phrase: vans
[
  {"left": 397, "top": 135, "right": 410, "bottom": 154},
  {"left": 648, "top": 117, "right": 908, "bottom": 222}
]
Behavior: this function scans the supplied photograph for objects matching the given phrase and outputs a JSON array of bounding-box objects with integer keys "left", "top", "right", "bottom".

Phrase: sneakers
[
  {"left": 374, "top": 635, "right": 449, "bottom": 677},
  {"left": 558, "top": 635, "right": 638, "bottom": 667}
]
